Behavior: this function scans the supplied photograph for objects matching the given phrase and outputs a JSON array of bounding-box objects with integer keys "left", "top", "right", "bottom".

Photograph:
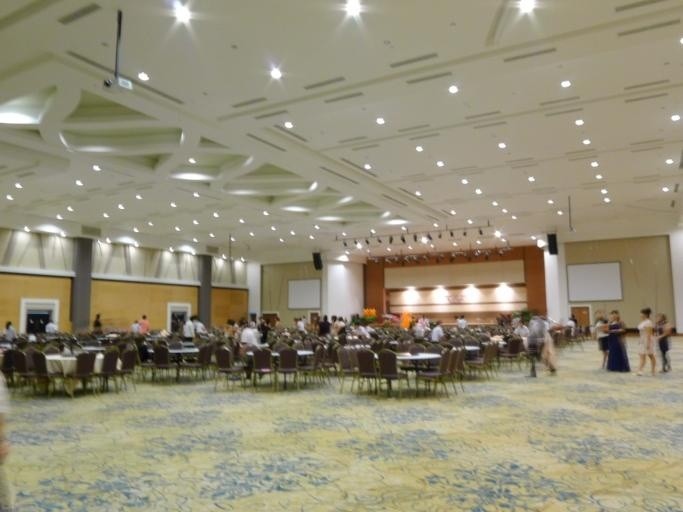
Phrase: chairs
[{"left": 0, "top": 325, "right": 592, "bottom": 400}]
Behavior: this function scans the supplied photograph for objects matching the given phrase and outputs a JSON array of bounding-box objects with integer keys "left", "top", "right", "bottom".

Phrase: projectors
[{"left": 103, "top": 79, "right": 133, "bottom": 95}]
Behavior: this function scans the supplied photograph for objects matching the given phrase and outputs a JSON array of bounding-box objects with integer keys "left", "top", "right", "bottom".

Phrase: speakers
[
  {"left": 312, "top": 252, "right": 323, "bottom": 271},
  {"left": 547, "top": 233, "right": 559, "bottom": 255}
]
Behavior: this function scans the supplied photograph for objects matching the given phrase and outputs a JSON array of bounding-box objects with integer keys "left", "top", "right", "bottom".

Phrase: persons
[
  {"left": 594, "top": 310, "right": 630, "bottom": 372},
  {"left": 637, "top": 309, "right": 656, "bottom": 375},
  {"left": 653, "top": 312, "right": 670, "bottom": 371},
  {"left": 183, "top": 309, "right": 589, "bottom": 378},
  {"left": 3, "top": 321, "right": 19, "bottom": 342},
  {"left": 94, "top": 314, "right": 102, "bottom": 335},
  {"left": 130, "top": 319, "right": 141, "bottom": 338},
  {"left": 0, "top": 368, "right": 14, "bottom": 512},
  {"left": 138, "top": 315, "right": 150, "bottom": 335},
  {"left": 46, "top": 318, "right": 59, "bottom": 334}
]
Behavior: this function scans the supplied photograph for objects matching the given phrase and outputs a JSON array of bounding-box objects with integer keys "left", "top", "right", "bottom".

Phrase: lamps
[{"left": 336, "top": 220, "right": 511, "bottom": 264}]
